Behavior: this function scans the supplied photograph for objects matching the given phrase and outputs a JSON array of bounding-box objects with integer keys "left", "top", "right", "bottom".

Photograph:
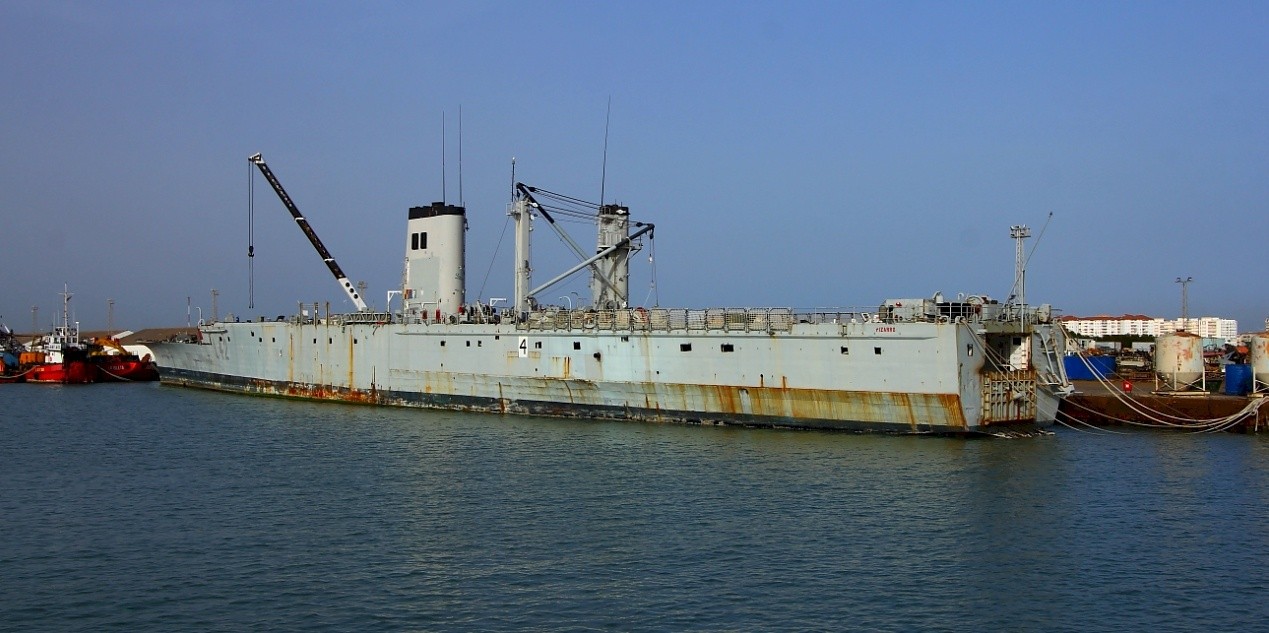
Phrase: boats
[
  {"left": 0, "top": 330, "right": 149, "bottom": 383},
  {"left": 158, "top": 324, "right": 1068, "bottom": 437}
]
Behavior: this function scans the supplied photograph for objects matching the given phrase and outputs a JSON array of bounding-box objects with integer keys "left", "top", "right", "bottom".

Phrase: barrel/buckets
[{"left": 1225, "top": 364, "right": 1251, "bottom": 395}]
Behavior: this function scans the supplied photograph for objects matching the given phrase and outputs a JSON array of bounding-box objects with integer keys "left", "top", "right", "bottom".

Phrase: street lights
[
  {"left": 1010, "top": 223, "right": 1031, "bottom": 318},
  {"left": 1176, "top": 277, "right": 1195, "bottom": 335}
]
